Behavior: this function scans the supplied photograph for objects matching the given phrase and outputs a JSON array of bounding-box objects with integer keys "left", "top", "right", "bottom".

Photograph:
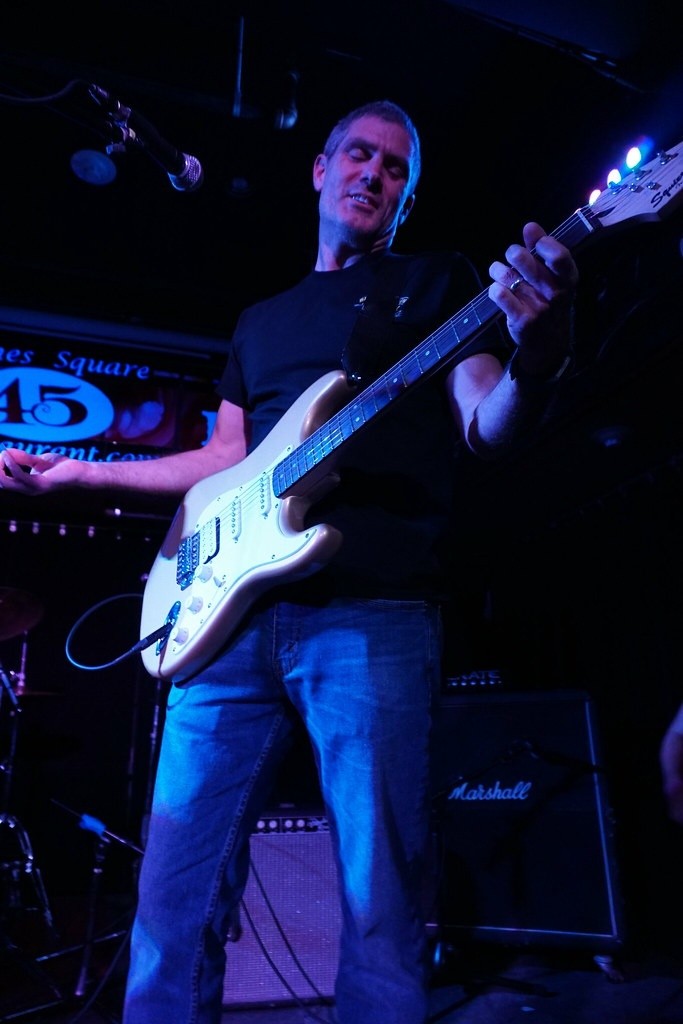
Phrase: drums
[{"left": 0, "top": 809, "right": 55, "bottom": 933}]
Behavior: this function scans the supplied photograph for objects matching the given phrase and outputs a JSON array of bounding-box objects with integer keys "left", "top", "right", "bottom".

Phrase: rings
[{"left": 510, "top": 277, "right": 523, "bottom": 291}]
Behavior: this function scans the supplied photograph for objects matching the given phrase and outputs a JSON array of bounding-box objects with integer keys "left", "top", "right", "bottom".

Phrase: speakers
[
  {"left": 215, "top": 813, "right": 346, "bottom": 1003},
  {"left": 431, "top": 688, "right": 625, "bottom": 956}
]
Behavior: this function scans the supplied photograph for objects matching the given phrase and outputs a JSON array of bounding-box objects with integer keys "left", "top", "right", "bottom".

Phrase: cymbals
[
  {"left": 0, "top": 585, "right": 48, "bottom": 643},
  {"left": 2, "top": 682, "right": 67, "bottom": 701}
]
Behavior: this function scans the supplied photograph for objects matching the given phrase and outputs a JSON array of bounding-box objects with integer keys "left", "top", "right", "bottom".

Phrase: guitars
[{"left": 137, "top": 138, "right": 683, "bottom": 688}]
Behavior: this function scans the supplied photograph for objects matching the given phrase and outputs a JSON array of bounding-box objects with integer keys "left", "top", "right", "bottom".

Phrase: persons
[
  {"left": 660, "top": 703, "right": 683, "bottom": 823},
  {"left": 0, "top": 96, "right": 582, "bottom": 1024}
]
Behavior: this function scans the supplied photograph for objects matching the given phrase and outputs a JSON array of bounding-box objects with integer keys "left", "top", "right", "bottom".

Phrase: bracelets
[{"left": 508, "top": 331, "right": 575, "bottom": 380}]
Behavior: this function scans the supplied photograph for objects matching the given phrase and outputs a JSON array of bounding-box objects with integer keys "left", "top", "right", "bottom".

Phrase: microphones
[{"left": 88, "top": 83, "right": 203, "bottom": 193}]
